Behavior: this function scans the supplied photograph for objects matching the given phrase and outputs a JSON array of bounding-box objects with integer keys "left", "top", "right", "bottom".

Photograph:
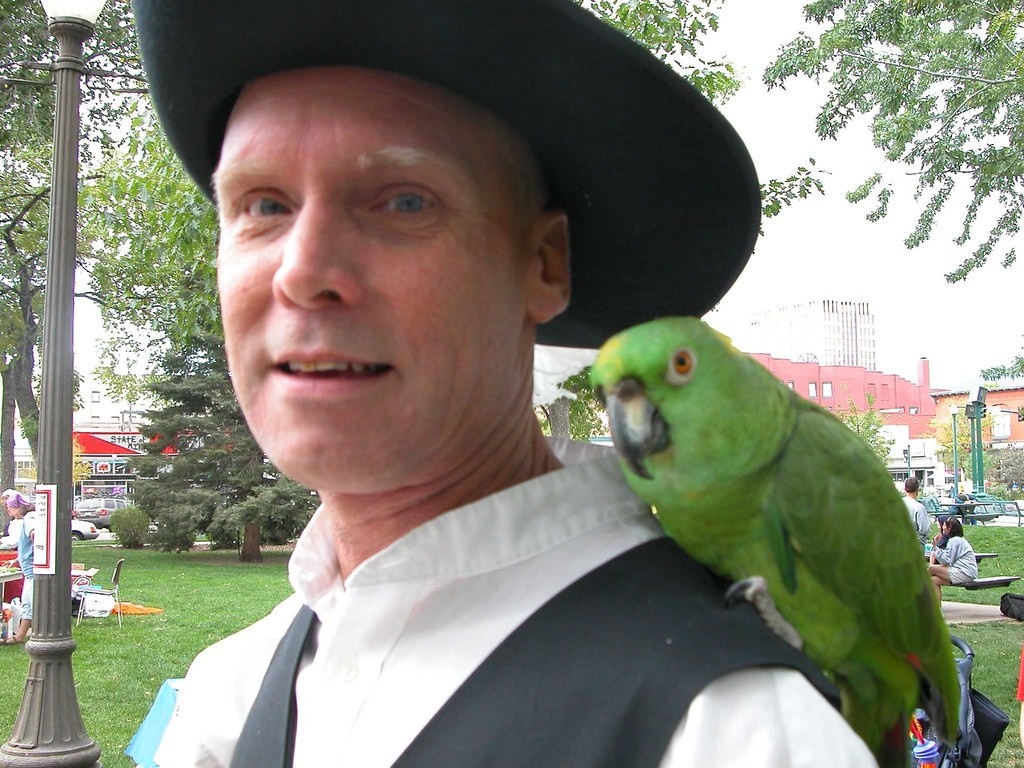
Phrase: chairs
[{"left": 76, "top": 558, "right": 124, "bottom": 628}]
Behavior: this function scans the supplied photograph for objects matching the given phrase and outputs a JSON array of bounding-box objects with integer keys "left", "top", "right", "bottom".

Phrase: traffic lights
[
  {"left": 980, "top": 405, "right": 986, "bottom": 418},
  {"left": 1018, "top": 406, "right": 1024, "bottom": 422}
]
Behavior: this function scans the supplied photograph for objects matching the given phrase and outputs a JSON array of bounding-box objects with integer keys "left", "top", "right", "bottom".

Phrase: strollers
[{"left": 910, "top": 633, "right": 974, "bottom": 768}]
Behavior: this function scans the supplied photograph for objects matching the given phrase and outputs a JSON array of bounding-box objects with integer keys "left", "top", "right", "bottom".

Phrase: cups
[{"left": 925, "top": 544, "right": 933, "bottom": 555}]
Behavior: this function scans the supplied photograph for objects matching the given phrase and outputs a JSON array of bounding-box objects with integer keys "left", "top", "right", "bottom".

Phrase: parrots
[{"left": 588, "top": 313, "right": 963, "bottom": 752}]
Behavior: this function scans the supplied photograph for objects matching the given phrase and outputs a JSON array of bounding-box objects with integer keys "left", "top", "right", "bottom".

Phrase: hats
[{"left": 131, "top": 0, "right": 762, "bottom": 350}]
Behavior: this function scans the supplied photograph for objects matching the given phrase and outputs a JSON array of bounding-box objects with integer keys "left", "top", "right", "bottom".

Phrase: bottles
[{"left": 2, "top": 623, "right": 7, "bottom": 639}]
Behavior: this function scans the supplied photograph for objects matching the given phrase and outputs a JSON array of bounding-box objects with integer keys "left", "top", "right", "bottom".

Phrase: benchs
[
  {"left": 992, "top": 501, "right": 1024, "bottom": 527},
  {"left": 915, "top": 498, "right": 949, "bottom": 521},
  {"left": 948, "top": 504, "right": 996, "bottom": 523},
  {"left": 919, "top": 495, "right": 945, "bottom": 511},
  {"left": 974, "top": 494, "right": 995, "bottom": 504},
  {"left": 958, "top": 576, "right": 1021, "bottom": 591},
  {"left": 964, "top": 513, "right": 1003, "bottom": 526},
  {"left": 930, "top": 513, "right": 962, "bottom": 524}
]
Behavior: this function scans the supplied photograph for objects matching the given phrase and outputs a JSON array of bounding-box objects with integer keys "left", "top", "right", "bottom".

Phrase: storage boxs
[{"left": 70, "top": 564, "right": 99, "bottom": 590}]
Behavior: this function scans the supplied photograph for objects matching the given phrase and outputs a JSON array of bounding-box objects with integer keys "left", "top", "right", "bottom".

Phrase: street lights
[
  {"left": 0, "top": 0, "right": 110, "bottom": 766},
  {"left": 950, "top": 402, "right": 959, "bottom": 500}
]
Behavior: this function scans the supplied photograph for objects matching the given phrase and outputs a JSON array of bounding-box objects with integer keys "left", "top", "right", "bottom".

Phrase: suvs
[{"left": 75, "top": 498, "right": 135, "bottom": 533}]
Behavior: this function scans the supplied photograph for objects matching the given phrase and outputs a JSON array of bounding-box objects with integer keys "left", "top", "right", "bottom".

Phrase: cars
[{"left": 72, "top": 520, "right": 100, "bottom": 542}]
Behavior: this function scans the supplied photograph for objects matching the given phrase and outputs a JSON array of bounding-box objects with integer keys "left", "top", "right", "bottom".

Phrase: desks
[
  {"left": 925, "top": 553, "right": 998, "bottom": 566},
  {"left": 0, "top": 565, "right": 23, "bottom": 583},
  {"left": 939, "top": 503, "right": 994, "bottom": 526}
]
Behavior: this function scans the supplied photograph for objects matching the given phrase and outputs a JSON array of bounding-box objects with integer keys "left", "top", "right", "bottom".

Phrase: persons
[
  {"left": 130, "top": 0, "right": 878, "bottom": 768},
  {"left": 0, "top": 489, "right": 35, "bottom": 645},
  {"left": 902, "top": 477, "right": 978, "bottom": 618}
]
